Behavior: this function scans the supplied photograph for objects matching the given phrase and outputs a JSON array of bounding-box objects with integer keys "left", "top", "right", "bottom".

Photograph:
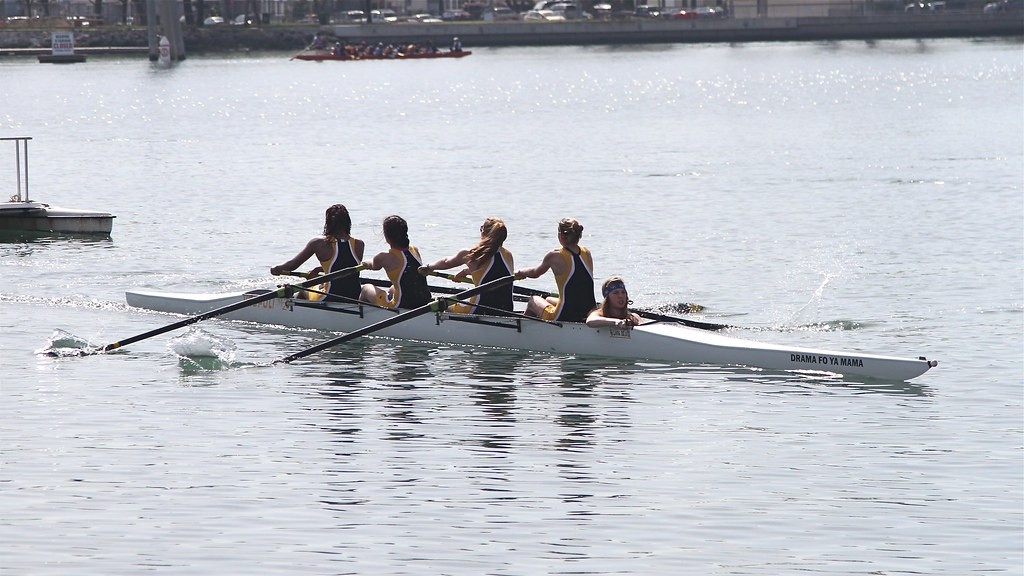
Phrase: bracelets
[{"left": 426, "top": 264, "right": 434, "bottom": 271}]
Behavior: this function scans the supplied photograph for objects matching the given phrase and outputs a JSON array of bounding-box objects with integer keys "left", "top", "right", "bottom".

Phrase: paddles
[
  {"left": 273, "top": 274, "right": 514, "bottom": 364},
  {"left": 282, "top": 269, "right": 867, "bottom": 332},
  {"left": 78, "top": 266, "right": 368, "bottom": 355},
  {"left": 429, "top": 269, "right": 706, "bottom": 315}
]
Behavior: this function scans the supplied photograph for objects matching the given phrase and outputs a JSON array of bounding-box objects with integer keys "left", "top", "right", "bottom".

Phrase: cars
[
  {"left": 903, "top": 1, "right": 1009, "bottom": 13},
  {"left": 6, "top": 0, "right": 726, "bottom": 30}
]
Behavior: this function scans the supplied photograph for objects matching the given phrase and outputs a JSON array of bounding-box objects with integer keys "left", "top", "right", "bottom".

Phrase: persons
[
  {"left": 309, "top": 32, "right": 324, "bottom": 49},
  {"left": 332, "top": 40, "right": 434, "bottom": 56},
  {"left": 418, "top": 216, "right": 514, "bottom": 315},
  {"left": 515, "top": 218, "right": 597, "bottom": 322},
  {"left": 586, "top": 276, "right": 643, "bottom": 328},
  {"left": 453, "top": 38, "right": 462, "bottom": 51},
  {"left": 358, "top": 215, "right": 431, "bottom": 309},
  {"left": 272, "top": 204, "right": 365, "bottom": 303}
]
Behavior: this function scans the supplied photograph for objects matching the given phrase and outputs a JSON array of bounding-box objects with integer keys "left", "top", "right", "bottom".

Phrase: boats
[
  {"left": 124, "top": 290, "right": 938, "bottom": 385},
  {"left": 297, "top": 51, "right": 472, "bottom": 60}
]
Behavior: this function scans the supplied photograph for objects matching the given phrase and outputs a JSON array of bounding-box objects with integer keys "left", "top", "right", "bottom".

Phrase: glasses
[{"left": 480, "top": 226, "right": 485, "bottom": 233}]
